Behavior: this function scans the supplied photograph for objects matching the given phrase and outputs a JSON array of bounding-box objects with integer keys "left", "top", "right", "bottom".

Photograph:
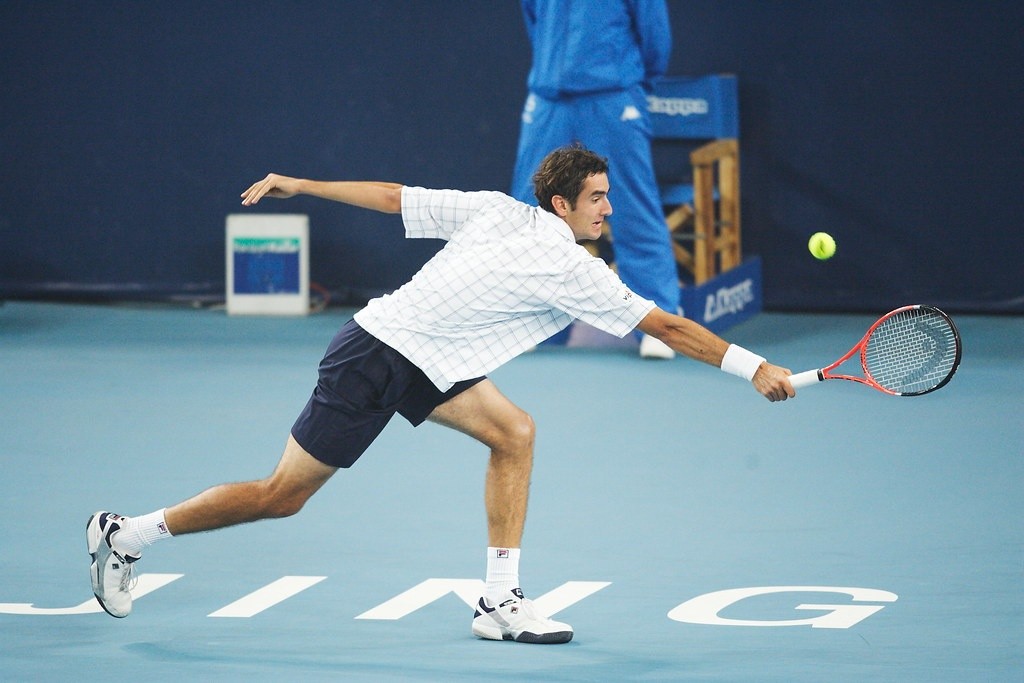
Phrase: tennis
[{"left": 808, "top": 232, "right": 836, "bottom": 260}]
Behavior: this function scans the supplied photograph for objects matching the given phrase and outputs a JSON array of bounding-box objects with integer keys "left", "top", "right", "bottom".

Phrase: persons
[
  {"left": 512, "top": 0, "right": 683, "bottom": 359},
  {"left": 83, "top": 143, "right": 795, "bottom": 645}
]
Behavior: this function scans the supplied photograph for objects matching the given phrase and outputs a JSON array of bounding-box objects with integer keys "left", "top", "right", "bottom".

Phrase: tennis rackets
[{"left": 787, "top": 305, "right": 962, "bottom": 397}]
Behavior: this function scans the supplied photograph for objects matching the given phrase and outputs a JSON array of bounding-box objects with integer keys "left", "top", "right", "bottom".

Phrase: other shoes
[{"left": 640, "top": 330, "right": 674, "bottom": 360}]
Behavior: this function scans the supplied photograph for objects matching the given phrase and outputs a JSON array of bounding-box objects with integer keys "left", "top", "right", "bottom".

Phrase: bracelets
[{"left": 720, "top": 344, "right": 766, "bottom": 382}]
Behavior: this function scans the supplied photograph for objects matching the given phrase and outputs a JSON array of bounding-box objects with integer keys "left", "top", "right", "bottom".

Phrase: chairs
[{"left": 581, "top": 74, "right": 741, "bottom": 289}]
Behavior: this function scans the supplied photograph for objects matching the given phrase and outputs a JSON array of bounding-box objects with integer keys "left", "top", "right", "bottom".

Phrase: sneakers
[
  {"left": 86, "top": 509, "right": 145, "bottom": 618},
  {"left": 469, "top": 589, "right": 573, "bottom": 642}
]
